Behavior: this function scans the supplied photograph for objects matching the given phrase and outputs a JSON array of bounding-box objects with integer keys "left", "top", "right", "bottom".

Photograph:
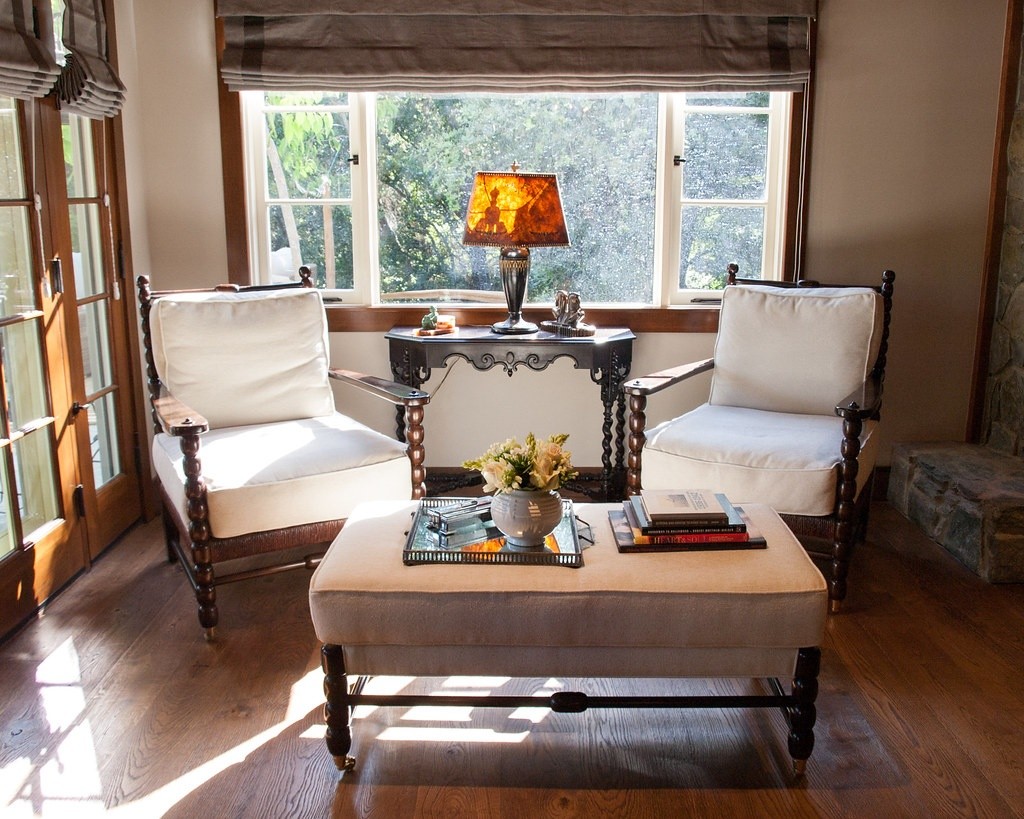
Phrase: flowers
[{"left": 460, "top": 432, "right": 580, "bottom": 495}]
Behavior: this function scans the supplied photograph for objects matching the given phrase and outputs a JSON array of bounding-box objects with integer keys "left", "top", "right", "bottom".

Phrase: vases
[{"left": 490, "top": 489, "right": 563, "bottom": 547}]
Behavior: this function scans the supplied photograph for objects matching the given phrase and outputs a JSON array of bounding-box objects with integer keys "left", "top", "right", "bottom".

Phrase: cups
[{"left": 436, "top": 314, "right": 455, "bottom": 329}]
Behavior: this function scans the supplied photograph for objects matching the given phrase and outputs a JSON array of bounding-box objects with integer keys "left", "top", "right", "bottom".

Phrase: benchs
[{"left": 308, "top": 501, "right": 829, "bottom": 782}]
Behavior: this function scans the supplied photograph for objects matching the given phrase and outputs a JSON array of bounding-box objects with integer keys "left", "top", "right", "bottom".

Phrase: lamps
[{"left": 463, "top": 160, "right": 572, "bottom": 334}]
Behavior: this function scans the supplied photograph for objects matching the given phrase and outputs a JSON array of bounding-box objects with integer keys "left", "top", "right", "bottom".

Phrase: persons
[{"left": 421, "top": 306, "right": 439, "bottom": 330}]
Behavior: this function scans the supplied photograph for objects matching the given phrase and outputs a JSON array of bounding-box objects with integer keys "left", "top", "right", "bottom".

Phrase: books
[{"left": 609, "top": 490, "right": 766, "bottom": 553}]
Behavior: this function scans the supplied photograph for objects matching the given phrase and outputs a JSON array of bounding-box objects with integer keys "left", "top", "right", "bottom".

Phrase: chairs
[
  {"left": 623, "top": 263, "right": 897, "bottom": 613},
  {"left": 136, "top": 265, "right": 431, "bottom": 644}
]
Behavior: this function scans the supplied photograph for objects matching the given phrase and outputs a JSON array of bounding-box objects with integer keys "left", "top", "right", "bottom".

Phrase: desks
[{"left": 382, "top": 323, "right": 636, "bottom": 501}]
